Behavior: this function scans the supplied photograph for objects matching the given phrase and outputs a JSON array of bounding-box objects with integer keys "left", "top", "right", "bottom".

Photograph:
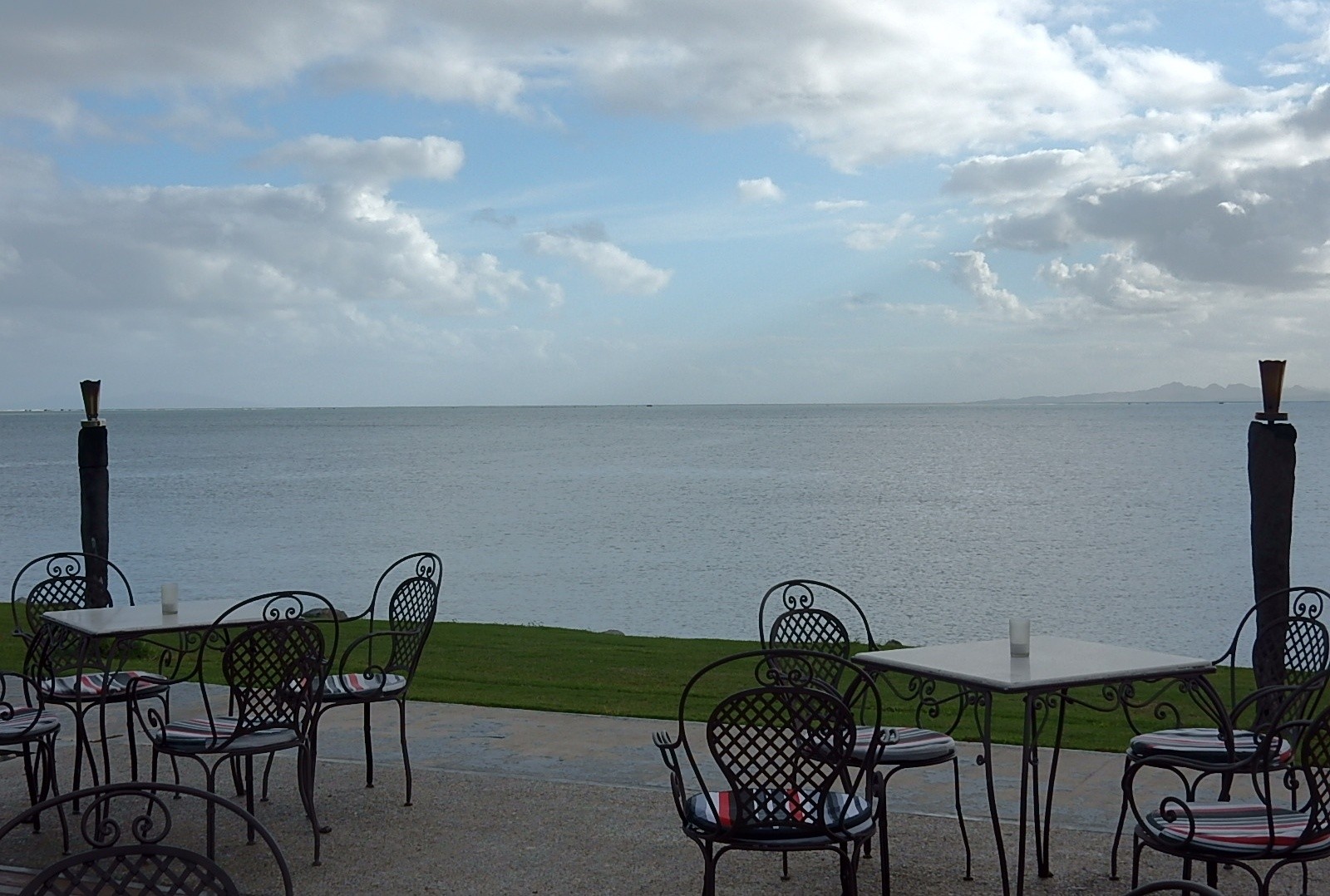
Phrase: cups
[
  {"left": 1009, "top": 617, "right": 1030, "bottom": 658},
  {"left": 161, "top": 583, "right": 177, "bottom": 615}
]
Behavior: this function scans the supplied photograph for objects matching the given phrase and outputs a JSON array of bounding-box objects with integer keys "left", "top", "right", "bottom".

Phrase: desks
[
  {"left": 43, "top": 598, "right": 305, "bottom": 814},
  {"left": 852, "top": 633, "right": 1217, "bottom": 896}
]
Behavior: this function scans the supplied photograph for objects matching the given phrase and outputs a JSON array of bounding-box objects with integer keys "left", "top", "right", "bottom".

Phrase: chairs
[
  {"left": 0, "top": 550, "right": 443, "bottom": 896},
  {"left": 653, "top": 580, "right": 1330, "bottom": 896}
]
